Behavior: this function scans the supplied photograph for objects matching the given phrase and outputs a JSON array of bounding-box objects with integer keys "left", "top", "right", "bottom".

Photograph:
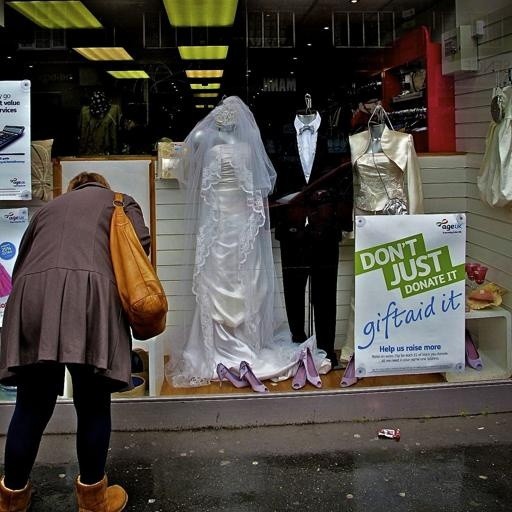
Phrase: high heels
[{"left": 217, "top": 361, "right": 269, "bottom": 392}]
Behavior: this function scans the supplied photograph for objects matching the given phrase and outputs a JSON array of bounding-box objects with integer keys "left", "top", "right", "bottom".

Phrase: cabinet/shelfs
[
  {"left": 382, "top": 27, "right": 456, "bottom": 152},
  {"left": 351, "top": 55, "right": 383, "bottom": 132},
  {"left": 443, "top": 303, "right": 511, "bottom": 383}
]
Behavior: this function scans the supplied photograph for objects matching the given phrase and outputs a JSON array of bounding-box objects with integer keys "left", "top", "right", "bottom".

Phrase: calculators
[{"left": 0, "top": 126, "right": 25, "bottom": 151}]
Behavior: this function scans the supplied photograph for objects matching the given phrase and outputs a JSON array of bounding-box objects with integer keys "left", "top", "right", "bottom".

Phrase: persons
[
  {"left": 0, "top": 171, "right": 153, "bottom": 512},
  {"left": 263, "top": 108, "right": 353, "bottom": 370},
  {"left": 339, "top": 105, "right": 423, "bottom": 364},
  {"left": 162, "top": 96, "right": 327, "bottom": 389}
]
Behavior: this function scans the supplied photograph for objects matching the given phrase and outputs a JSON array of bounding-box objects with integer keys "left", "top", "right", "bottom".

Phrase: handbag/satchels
[{"left": 110, "top": 192, "right": 167, "bottom": 340}]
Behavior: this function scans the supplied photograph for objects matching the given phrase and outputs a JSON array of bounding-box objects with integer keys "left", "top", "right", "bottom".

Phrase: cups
[{"left": 472, "top": 265, "right": 489, "bottom": 286}]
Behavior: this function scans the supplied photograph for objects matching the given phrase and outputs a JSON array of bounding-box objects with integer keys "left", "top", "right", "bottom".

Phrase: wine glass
[{"left": 465, "top": 262, "right": 479, "bottom": 291}]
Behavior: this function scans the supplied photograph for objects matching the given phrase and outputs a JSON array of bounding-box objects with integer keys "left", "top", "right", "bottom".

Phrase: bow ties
[{"left": 299, "top": 126, "right": 314, "bottom": 135}]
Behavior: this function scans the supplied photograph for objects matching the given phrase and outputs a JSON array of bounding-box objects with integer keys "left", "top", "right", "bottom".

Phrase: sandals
[
  {"left": 292, "top": 346, "right": 322, "bottom": 389},
  {"left": 340, "top": 353, "right": 357, "bottom": 388},
  {"left": 466, "top": 330, "right": 483, "bottom": 371}
]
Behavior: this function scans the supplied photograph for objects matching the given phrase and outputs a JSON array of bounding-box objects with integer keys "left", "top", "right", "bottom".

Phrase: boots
[
  {"left": 0, "top": 475, "right": 31, "bottom": 512},
  {"left": 75, "top": 474, "right": 128, "bottom": 512}
]
